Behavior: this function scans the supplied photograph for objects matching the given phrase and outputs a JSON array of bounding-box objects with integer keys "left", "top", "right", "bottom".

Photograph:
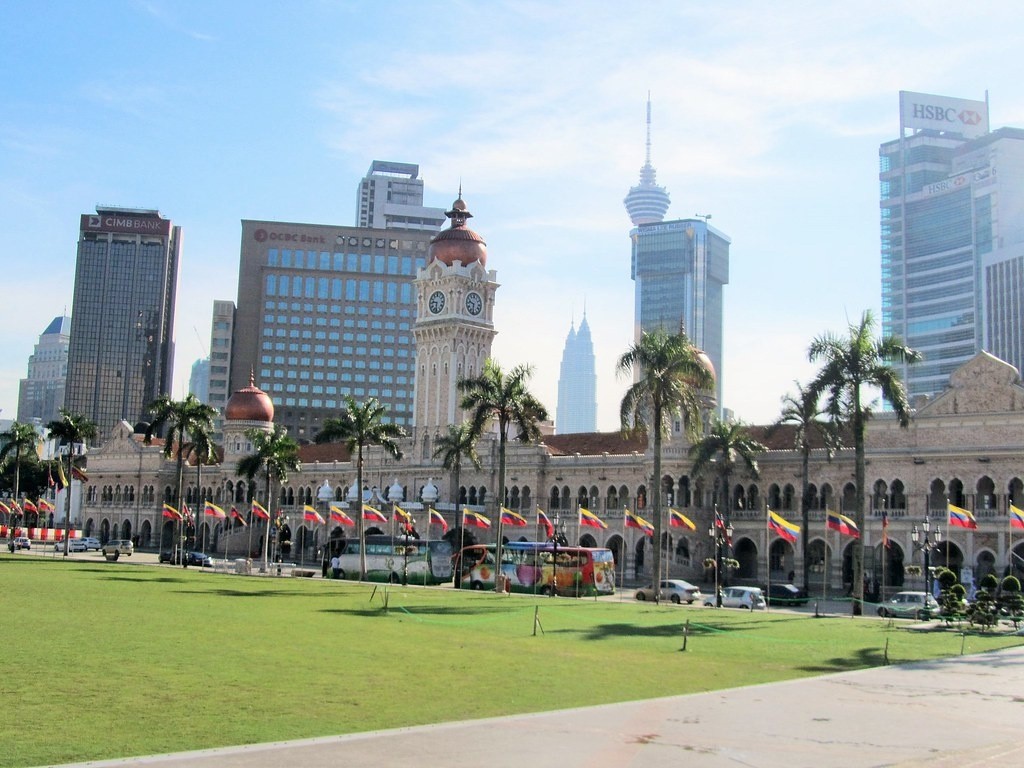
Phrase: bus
[
  {"left": 449, "top": 542, "right": 616, "bottom": 598},
  {"left": 320, "top": 534, "right": 454, "bottom": 587}
]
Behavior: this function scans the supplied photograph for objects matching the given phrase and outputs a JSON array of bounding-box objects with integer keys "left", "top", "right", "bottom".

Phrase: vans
[{"left": 102, "top": 539, "right": 134, "bottom": 556}]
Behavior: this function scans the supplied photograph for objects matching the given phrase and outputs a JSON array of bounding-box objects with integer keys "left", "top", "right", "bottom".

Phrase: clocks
[
  {"left": 428, "top": 291, "right": 445, "bottom": 314},
  {"left": 466, "top": 293, "right": 482, "bottom": 315}
]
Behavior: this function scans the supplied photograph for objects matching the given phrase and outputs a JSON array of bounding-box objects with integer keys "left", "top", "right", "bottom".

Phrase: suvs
[{"left": 8, "top": 537, "right": 31, "bottom": 551}]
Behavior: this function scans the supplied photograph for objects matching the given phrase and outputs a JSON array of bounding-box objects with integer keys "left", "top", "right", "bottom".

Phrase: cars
[
  {"left": 54, "top": 539, "right": 86, "bottom": 552},
  {"left": 761, "top": 584, "right": 808, "bottom": 607},
  {"left": 634, "top": 579, "right": 702, "bottom": 605},
  {"left": 876, "top": 590, "right": 941, "bottom": 620},
  {"left": 702, "top": 586, "right": 766, "bottom": 610},
  {"left": 78, "top": 537, "right": 101, "bottom": 551},
  {"left": 159, "top": 550, "right": 213, "bottom": 567}
]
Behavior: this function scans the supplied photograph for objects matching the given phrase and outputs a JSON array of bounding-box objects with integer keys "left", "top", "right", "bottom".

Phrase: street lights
[
  {"left": 911, "top": 515, "right": 942, "bottom": 622},
  {"left": 550, "top": 513, "right": 566, "bottom": 597},
  {"left": 274, "top": 510, "right": 290, "bottom": 575},
  {"left": 183, "top": 507, "right": 196, "bottom": 568},
  {"left": 397, "top": 512, "right": 417, "bottom": 585},
  {"left": 708, "top": 513, "right": 734, "bottom": 608}
]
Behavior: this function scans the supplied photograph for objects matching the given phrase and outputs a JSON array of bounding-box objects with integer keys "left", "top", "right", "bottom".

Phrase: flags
[
  {"left": 715, "top": 512, "right": 732, "bottom": 546},
  {"left": 330, "top": 505, "right": 354, "bottom": 525},
  {"left": 304, "top": 505, "right": 326, "bottom": 525},
  {"left": 501, "top": 508, "right": 527, "bottom": 527},
  {"left": 948, "top": 505, "right": 977, "bottom": 529},
  {"left": 162, "top": 499, "right": 270, "bottom": 527},
  {"left": 363, "top": 505, "right": 387, "bottom": 523},
  {"left": 1009, "top": 505, "right": 1024, "bottom": 529},
  {"left": 883, "top": 510, "right": 891, "bottom": 548},
  {"left": 431, "top": 509, "right": 447, "bottom": 533},
  {"left": 0, "top": 497, "right": 55, "bottom": 517},
  {"left": 538, "top": 510, "right": 554, "bottom": 537},
  {"left": 393, "top": 506, "right": 412, "bottom": 530},
  {"left": 464, "top": 509, "right": 491, "bottom": 528},
  {"left": 768, "top": 509, "right": 799, "bottom": 542},
  {"left": 669, "top": 509, "right": 695, "bottom": 532},
  {"left": 579, "top": 508, "right": 607, "bottom": 529},
  {"left": 625, "top": 509, "right": 654, "bottom": 536},
  {"left": 49, "top": 455, "right": 69, "bottom": 494},
  {"left": 827, "top": 509, "right": 859, "bottom": 538}
]
